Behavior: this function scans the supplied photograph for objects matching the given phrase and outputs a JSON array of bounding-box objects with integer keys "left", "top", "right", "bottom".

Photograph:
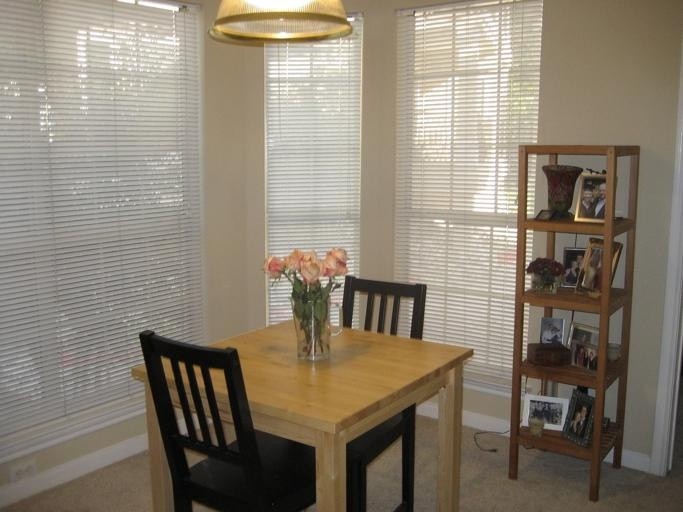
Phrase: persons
[{"left": 532, "top": 183, "right": 607, "bottom": 440}]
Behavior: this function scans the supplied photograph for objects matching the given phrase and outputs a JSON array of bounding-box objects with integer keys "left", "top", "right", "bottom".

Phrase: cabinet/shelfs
[{"left": 505, "top": 142, "right": 644, "bottom": 503}]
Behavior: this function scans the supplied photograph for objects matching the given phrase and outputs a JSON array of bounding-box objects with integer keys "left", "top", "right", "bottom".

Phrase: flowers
[
  {"left": 526, "top": 256, "right": 565, "bottom": 295},
  {"left": 259, "top": 246, "right": 349, "bottom": 357}
]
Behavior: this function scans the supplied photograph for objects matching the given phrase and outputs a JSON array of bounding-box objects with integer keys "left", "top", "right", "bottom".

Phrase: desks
[{"left": 129, "top": 319, "right": 474, "bottom": 512}]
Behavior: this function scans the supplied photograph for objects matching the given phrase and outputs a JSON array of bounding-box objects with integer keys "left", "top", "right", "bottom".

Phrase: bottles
[{"left": 528, "top": 410, "right": 544, "bottom": 438}]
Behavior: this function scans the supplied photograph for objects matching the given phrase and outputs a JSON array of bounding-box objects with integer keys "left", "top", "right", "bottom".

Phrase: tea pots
[{"left": 287, "top": 296, "right": 343, "bottom": 363}]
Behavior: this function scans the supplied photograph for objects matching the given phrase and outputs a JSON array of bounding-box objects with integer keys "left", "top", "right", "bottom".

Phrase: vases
[
  {"left": 290, "top": 296, "right": 333, "bottom": 363},
  {"left": 530, "top": 273, "right": 558, "bottom": 294},
  {"left": 541, "top": 164, "right": 583, "bottom": 222}
]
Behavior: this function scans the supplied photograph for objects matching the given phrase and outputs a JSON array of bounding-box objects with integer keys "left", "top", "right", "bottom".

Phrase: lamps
[{"left": 207, "top": 0, "right": 357, "bottom": 51}]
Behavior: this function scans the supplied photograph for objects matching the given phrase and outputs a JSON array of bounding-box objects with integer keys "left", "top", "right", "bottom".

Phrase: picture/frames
[
  {"left": 559, "top": 236, "right": 624, "bottom": 299},
  {"left": 539, "top": 315, "right": 600, "bottom": 374},
  {"left": 572, "top": 171, "right": 617, "bottom": 225},
  {"left": 519, "top": 387, "right": 595, "bottom": 448}
]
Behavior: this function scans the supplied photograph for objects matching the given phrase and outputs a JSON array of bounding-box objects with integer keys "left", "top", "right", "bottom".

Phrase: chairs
[
  {"left": 137, "top": 328, "right": 368, "bottom": 511},
  {"left": 338, "top": 276, "right": 427, "bottom": 512}
]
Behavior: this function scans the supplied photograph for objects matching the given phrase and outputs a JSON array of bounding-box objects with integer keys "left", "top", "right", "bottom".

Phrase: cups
[{"left": 607, "top": 344, "right": 621, "bottom": 360}]
